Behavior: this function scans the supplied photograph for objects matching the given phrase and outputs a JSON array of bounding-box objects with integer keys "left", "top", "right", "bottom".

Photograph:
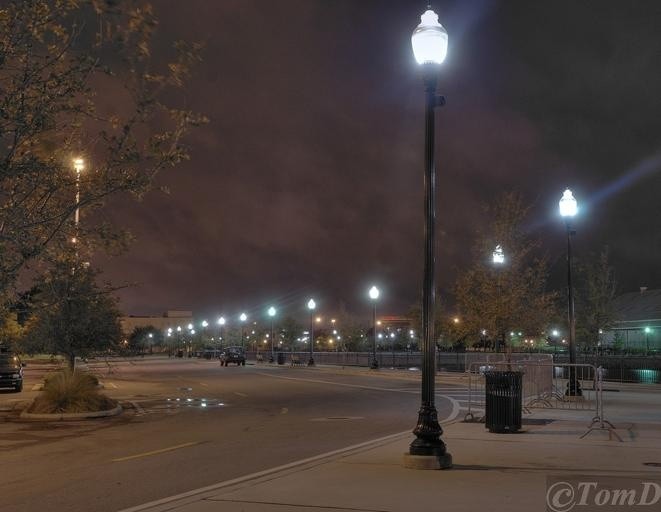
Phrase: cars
[{"left": 0, "top": 347, "right": 27, "bottom": 392}]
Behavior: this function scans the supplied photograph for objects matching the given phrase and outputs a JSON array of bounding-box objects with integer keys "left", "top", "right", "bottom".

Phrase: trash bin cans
[
  {"left": 483, "top": 371, "right": 523, "bottom": 433},
  {"left": 277, "top": 353, "right": 286, "bottom": 364}
]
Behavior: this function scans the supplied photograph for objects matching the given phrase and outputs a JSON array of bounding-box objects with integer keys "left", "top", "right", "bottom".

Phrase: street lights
[
  {"left": 493, "top": 244, "right": 504, "bottom": 355},
  {"left": 67, "top": 155, "right": 85, "bottom": 377},
  {"left": 147, "top": 286, "right": 382, "bottom": 373},
  {"left": 558, "top": 188, "right": 584, "bottom": 396},
  {"left": 400, "top": 0, "right": 452, "bottom": 470}
]
